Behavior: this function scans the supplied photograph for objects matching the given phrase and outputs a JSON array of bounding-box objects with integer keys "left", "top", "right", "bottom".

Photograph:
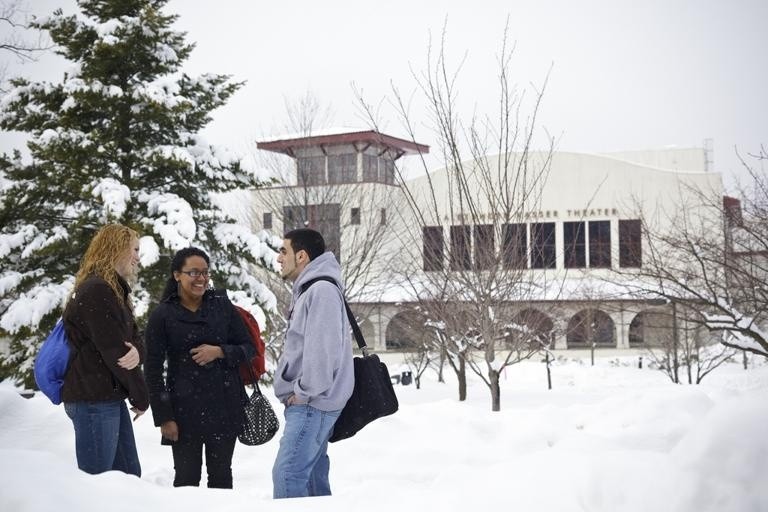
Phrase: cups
[{"left": 181, "top": 271, "right": 209, "bottom": 277}]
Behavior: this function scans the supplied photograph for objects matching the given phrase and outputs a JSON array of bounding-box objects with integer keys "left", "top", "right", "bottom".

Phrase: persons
[
  {"left": 143, "top": 246, "right": 257, "bottom": 489},
  {"left": 266, "top": 226, "right": 356, "bottom": 500},
  {"left": 58, "top": 222, "right": 146, "bottom": 478}
]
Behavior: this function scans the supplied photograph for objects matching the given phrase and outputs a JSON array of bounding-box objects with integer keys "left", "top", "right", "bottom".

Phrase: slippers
[{"left": 34, "top": 320, "right": 72, "bottom": 404}]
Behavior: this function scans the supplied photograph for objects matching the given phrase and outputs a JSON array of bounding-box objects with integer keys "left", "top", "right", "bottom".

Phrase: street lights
[
  {"left": 238, "top": 392, "right": 279, "bottom": 445},
  {"left": 215, "top": 288, "right": 264, "bottom": 385},
  {"left": 328, "top": 354, "right": 398, "bottom": 442}
]
